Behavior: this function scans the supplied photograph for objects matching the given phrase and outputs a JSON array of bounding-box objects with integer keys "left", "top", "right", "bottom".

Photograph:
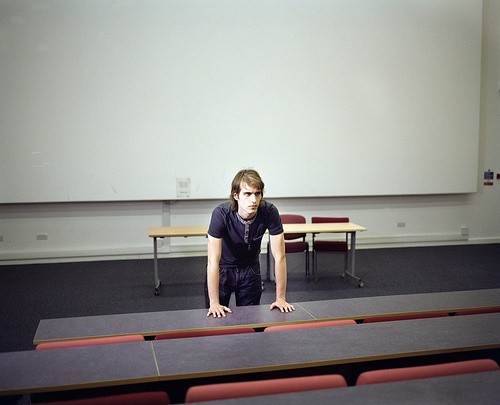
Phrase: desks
[{"left": 0, "top": 221, "right": 500, "bottom": 405}]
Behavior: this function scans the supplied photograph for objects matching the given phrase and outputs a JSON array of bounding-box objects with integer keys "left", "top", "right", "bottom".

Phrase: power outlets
[{"left": 398, "top": 221, "right": 406, "bottom": 227}]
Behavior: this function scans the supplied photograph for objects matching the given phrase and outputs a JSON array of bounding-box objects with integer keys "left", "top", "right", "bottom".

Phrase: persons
[{"left": 204, "top": 169, "right": 296, "bottom": 317}]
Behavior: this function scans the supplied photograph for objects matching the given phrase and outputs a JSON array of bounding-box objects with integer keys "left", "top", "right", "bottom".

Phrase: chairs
[
  {"left": 267, "top": 213, "right": 308, "bottom": 281},
  {"left": 312, "top": 216, "right": 350, "bottom": 282}
]
